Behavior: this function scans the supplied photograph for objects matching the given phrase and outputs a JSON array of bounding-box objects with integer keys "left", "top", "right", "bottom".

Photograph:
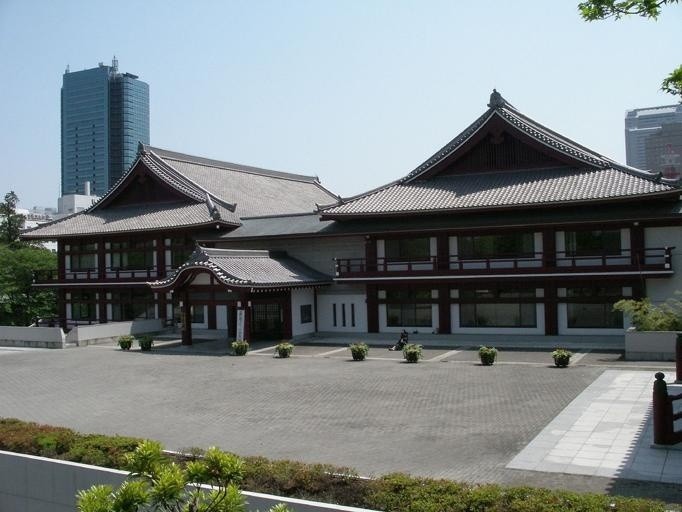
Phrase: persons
[{"left": 389, "top": 336, "right": 406, "bottom": 351}]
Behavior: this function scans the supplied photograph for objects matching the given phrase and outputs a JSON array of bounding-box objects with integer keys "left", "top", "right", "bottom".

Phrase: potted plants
[
  {"left": 231, "top": 338, "right": 294, "bottom": 358},
  {"left": 348, "top": 341, "right": 425, "bottom": 363},
  {"left": 117, "top": 333, "right": 155, "bottom": 352},
  {"left": 478, "top": 344, "right": 573, "bottom": 368}
]
